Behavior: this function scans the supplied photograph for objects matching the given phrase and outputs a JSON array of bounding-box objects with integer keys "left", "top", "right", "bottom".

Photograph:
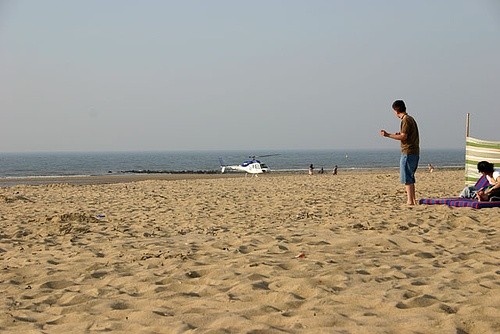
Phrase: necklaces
[{"left": 401, "top": 113, "right": 408, "bottom": 119}]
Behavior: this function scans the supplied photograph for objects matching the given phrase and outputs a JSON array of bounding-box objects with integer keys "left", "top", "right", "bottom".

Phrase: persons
[
  {"left": 476, "top": 161, "right": 500, "bottom": 204},
  {"left": 332, "top": 165, "right": 337, "bottom": 176},
  {"left": 427, "top": 164, "right": 434, "bottom": 172},
  {"left": 380, "top": 99, "right": 420, "bottom": 206},
  {"left": 309, "top": 164, "right": 314, "bottom": 175}
]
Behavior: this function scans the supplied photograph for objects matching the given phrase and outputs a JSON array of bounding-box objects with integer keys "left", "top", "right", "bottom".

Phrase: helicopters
[{"left": 219, "top": 153, "right": 285, "bottom": 178}]
